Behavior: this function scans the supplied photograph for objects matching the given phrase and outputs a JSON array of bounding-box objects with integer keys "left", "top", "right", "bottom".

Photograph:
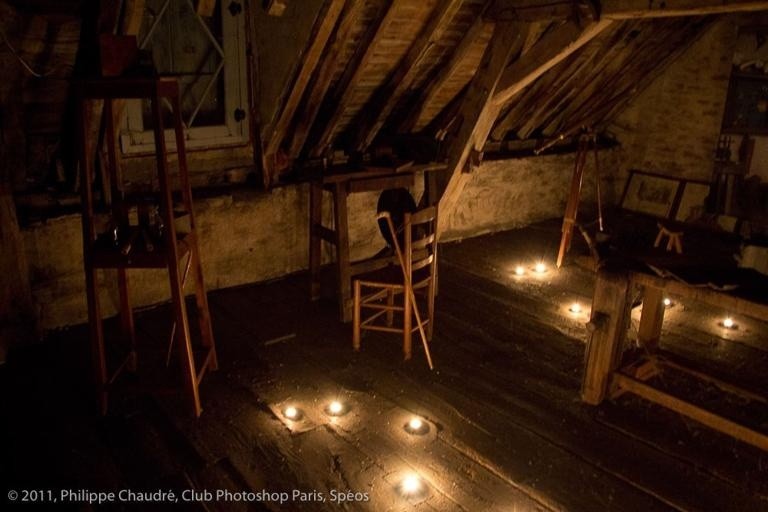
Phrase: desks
[{"left": 306, "top": 161, "right": 448, "bottom": 323}]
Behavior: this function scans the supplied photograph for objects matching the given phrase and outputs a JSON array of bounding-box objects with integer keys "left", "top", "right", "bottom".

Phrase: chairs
[{"left": 345, "top": 202, "right": 439, "bottom": 362}]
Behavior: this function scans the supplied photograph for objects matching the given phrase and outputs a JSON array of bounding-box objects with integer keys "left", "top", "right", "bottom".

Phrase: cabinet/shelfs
[{"left": 70, "top": 73, "right": 219, "bottom": 421}]
